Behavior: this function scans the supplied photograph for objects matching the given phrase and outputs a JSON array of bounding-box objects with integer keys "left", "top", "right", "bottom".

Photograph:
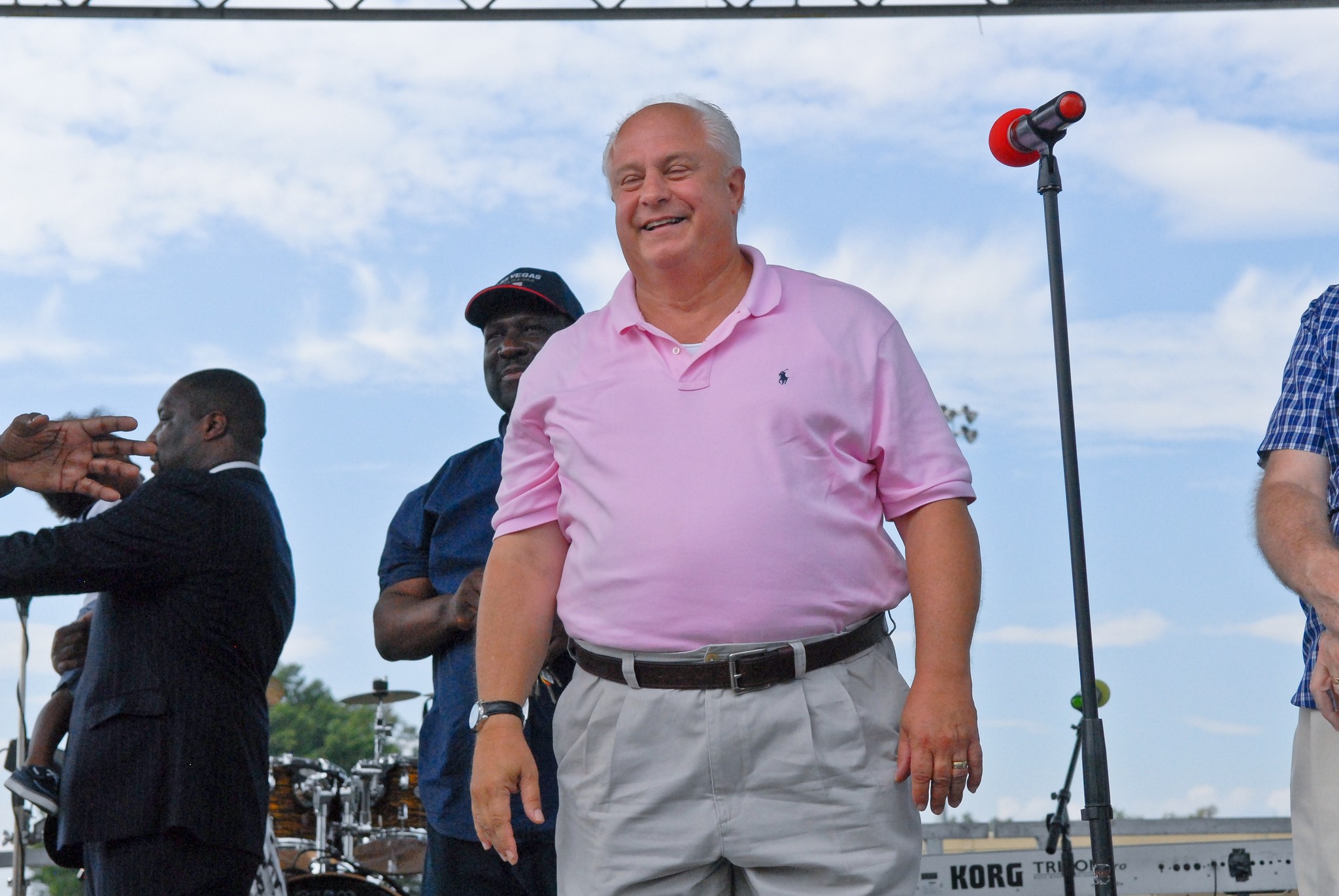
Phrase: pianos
[{"left": 914, "top": 837, "right": 1297, "bottom": 896}]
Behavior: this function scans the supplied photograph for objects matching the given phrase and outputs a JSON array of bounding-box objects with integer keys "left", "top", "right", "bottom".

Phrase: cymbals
[
  {"left": 339, "top": 689, "right": 421, "bottom": 706},
  {"left": 264, "top": 675, "right": 286, "bottom": 707}
]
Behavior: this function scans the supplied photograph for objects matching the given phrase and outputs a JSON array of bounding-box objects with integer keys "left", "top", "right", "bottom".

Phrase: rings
[
  {"left": 1332, "top": 678, "right": 1339, "bottom": 685},
  {"left": 952, "top": 761, "right": 968, "bottom": 769}
]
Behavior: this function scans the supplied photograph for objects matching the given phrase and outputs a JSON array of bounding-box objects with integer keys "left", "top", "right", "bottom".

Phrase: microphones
[
  {"left": 1070, "top": 679, "right": 1111, "bottom": 710},
  {"left": 988, "top": 91, "right": 1086, "bottom": 168}
]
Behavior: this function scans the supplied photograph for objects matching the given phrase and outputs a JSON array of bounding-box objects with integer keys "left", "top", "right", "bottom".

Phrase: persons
[
  {"left": 373, "top": 268, "right": 586, "bottom": 896},
  {"left": 0, "top": 368, "right": 295, "bottom": 896},
  {"left": 3, "top": 409, "right": 146, "bottom": 813},
  {"left": 0, "top": 413, "right": 158, "bottom": 502},
  {"left": 1255, "top": 284, "right": 1339, "bottom": 895},
  {"left": 469, "top": 97, "right": 982, "bottom": 896}
]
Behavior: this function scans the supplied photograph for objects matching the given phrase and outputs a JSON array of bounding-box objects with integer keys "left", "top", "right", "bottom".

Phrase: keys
[{"left": 534, "top": 665, "right": 563, "bottom": 704}]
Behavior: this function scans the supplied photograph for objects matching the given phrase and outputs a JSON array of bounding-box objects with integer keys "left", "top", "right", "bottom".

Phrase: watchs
[{"left": 469, "top": 699, "right": 524, "bottom": 733}]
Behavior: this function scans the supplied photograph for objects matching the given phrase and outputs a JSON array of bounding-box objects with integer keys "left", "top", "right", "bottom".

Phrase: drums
[
  {"left": 266, "top": 754, "right": 357, "bottom": 872},
  {"left": 349, "top": 753, "right": 429, "bottom": 876},
  {"left": 283, "top": 864, "right": 408, "bottom": 896}
]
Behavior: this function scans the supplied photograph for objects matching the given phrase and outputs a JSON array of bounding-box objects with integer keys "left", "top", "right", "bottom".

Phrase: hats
[{"left": 464, "top": 265, "right": 585, "bottom": 327}]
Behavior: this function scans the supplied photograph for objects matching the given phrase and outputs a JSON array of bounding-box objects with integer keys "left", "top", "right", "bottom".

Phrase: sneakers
[{"left": 4, "top": 764, "right": 60, "bottom": 818}]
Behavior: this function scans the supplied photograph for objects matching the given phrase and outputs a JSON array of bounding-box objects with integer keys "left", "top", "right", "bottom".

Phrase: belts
[{"left": 572, "top": 612, "right": 891, "bottom": 695}]
